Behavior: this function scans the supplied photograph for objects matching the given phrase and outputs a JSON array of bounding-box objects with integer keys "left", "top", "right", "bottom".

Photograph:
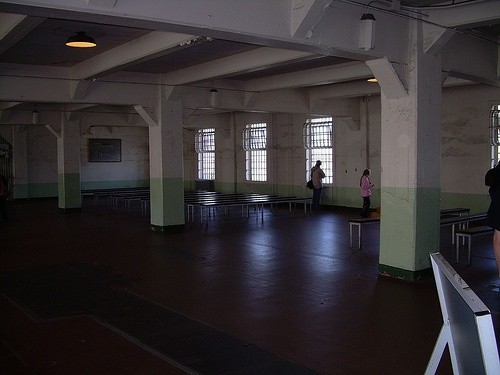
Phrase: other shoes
[{"left": 488, "top": 279, "right": 500, "bottom": 292}]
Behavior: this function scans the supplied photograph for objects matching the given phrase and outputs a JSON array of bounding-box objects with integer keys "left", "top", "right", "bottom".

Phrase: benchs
[
  {"left": 348, "top": 208, "right": 495, "bottom": 265},
  {"left": 93, "top": 188, "right": 312, "bottom": 228}
]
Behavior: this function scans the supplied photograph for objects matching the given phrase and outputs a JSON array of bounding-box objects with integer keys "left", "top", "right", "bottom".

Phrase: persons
[
  {"left": 360, "top": 169, "right": 374, "bottom": 213},
  {"left": 485, "top": 160, "right": 500, "bottom": 226},
  {"left": 311, "top": 160, "right": 325, "bottom": 210}
]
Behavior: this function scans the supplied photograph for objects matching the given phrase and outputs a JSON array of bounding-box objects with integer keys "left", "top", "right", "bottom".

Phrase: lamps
[{"left": 65, "top": 31, "right": 97, "bottom": 48}]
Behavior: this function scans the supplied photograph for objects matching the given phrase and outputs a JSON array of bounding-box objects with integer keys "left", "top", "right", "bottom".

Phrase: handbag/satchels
[{"left": 306, "top": 181, "right": 313, "bottom": 190}]
